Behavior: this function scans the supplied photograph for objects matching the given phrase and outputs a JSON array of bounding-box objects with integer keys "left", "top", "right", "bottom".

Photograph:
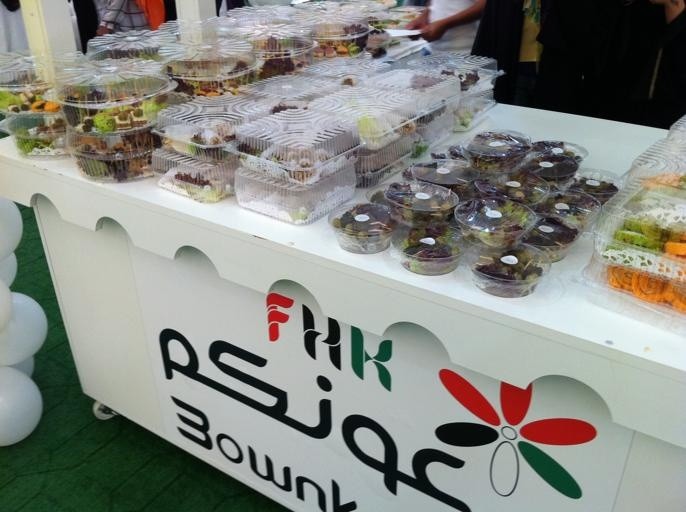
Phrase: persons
[
  {"left": 94, "top": 0, "right": 150, "bottom": 35},
  {"left": 592, "top": 0, "right": 686, "bottom": 128},
  {"left": 402, "top": 0, "right": 486, "bottom": 55}
]
